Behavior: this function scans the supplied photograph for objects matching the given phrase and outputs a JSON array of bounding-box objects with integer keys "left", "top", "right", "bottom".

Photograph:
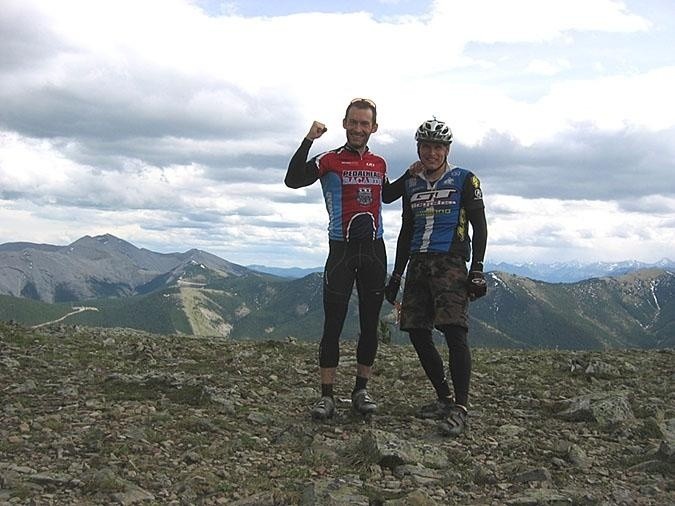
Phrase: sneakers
[
  {"left": 312, "top": 395, "right": 337, "bottom": 419},
  {"left": 416, "top": 398, "right": 455, "bottom": 418},
  {"left": 351, "top": 386, "right": 378, "bottom": 415},
  {"left": 437, "top": 405, "right": 467, "bottom": 435}
]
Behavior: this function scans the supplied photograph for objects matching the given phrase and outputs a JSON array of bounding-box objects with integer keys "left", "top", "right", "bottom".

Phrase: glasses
[{"left": 350, "top": 98, "right": 376, "bottom": 123}]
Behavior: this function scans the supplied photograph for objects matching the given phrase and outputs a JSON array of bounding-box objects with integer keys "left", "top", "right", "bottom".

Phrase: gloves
[
  {"left": 384, "top": 274, "right": 400, "bottom": 307},
  {"left": 466, "top": 270, "right": 487, "bottom": 301}
]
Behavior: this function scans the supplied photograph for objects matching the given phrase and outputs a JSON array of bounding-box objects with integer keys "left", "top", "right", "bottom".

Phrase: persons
[
  {"left": 283, "top": 95, "right": 423, "bottom": 421},
  {"left": 384, "top": 116, "right": 487, "bottom": 436}
]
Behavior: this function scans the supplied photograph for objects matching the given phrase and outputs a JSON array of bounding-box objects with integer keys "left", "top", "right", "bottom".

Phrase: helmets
[{"left": 415, "top": 120, "right": 453, "bottom": 144}]
